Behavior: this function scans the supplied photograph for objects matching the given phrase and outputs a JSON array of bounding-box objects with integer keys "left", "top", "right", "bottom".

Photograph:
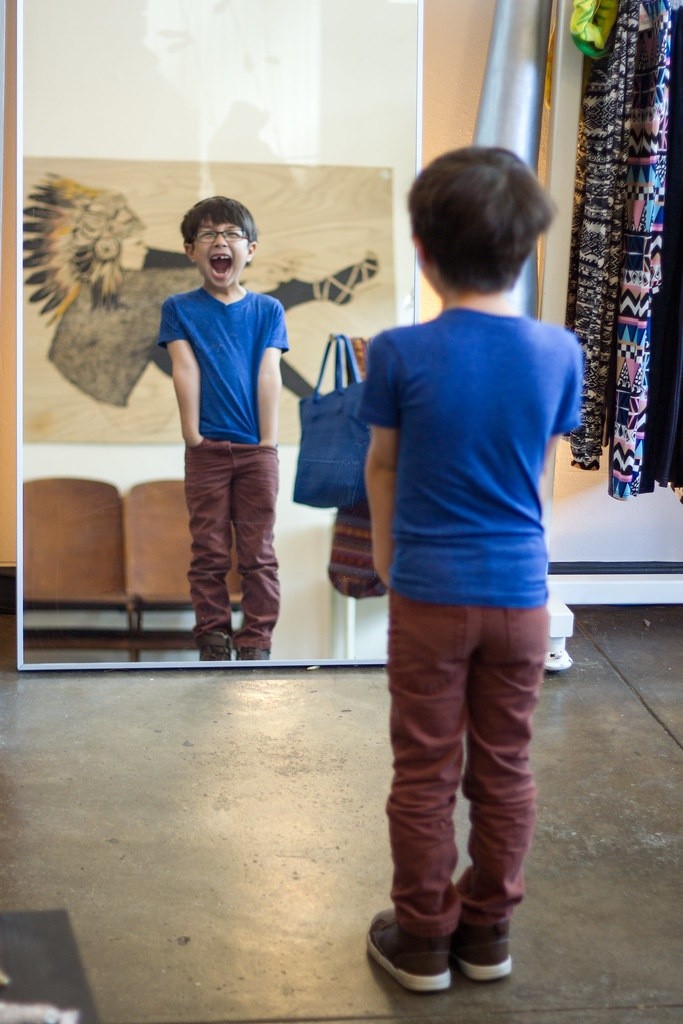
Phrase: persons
[
  {"left": 356, "top": 144, "right": 584, "bottom": 995},
  {"left": 158, "top": 196, "right": 290, "bottom": 663}
]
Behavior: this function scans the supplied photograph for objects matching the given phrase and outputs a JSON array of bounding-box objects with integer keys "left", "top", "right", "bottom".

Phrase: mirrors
[{"left": 9, "top": 2, "right": 423, "bottom": 673}]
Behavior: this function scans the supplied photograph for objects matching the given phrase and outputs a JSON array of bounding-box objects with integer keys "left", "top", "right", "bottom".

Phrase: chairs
[
  {"left": 124, "top": 477, "right": 246, "bottom": 661},
  {"left": 20, "top": 476, "right": 131, "bottom": 659}
]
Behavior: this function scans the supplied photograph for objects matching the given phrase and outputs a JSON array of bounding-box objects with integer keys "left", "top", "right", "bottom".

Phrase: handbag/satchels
[
  {"left": 294, "top": 335, "right": 372, "bottom": 510},
  {"left": 328, "top": 497, "right": 389, "bottom": 598}
]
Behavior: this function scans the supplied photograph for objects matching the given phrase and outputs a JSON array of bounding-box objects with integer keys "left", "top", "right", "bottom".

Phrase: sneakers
[
  {"left": 446, "top": 919, "right": 514, "bottom": 981},
  {"left": 236, "top": 647, "right": 269, "bottom": 659},
  {"left": 200, "top": 645, "right": 231, "bottom": 661},
  {"left": 366, "top": 910, "right": 451, "bottom": 992}
]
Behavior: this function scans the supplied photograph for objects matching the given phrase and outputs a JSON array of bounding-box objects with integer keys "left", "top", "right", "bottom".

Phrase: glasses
[{"left": 192, "top": 230, "right": 249, "bottom": 243}]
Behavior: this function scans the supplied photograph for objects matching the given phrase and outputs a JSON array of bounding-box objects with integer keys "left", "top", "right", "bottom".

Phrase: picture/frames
[{"left": 20, "top": 156, "right": 393, "bottom": 445}]
